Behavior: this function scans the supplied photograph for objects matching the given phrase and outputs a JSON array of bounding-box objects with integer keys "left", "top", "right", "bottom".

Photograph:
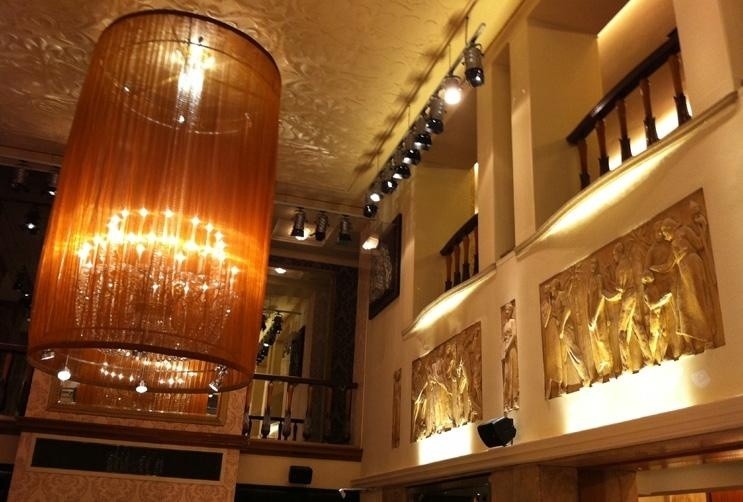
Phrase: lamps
[
  {"left": 363, "top": 41, "right": 483, "bottom": 216},
  {"left": 24, "top": 10, "right": 282, "bottom": 394},
  {"left": 291, "top": 208, "right": 352, "bottom": 243}
]
